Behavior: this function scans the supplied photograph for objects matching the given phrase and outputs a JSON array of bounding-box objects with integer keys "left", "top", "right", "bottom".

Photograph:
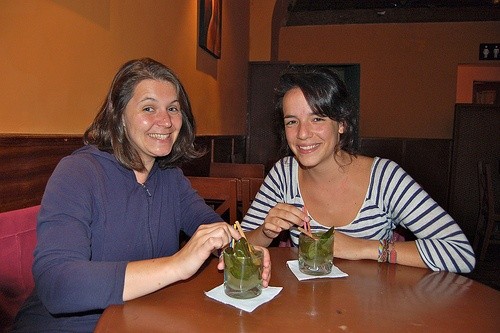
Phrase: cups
[
  {"left": 223, "top": 249, "right": 264, "bottom": 298},
  {"left": 299, "top": 234, "right": 334, "bottom": 274}
]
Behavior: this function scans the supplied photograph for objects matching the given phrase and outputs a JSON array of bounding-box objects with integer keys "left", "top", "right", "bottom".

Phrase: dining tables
[{"left": 93, "top": 247, "right": 500, "bottom": 333}]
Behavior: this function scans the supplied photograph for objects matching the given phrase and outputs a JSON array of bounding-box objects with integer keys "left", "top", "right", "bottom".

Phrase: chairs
[
  {"left": 241, "top": 178, "right": 264, "bottom": 220},
  {"left": 0, "top": 205, "right": 42, "bottom": 333},
  {"left": 179, "top": 177, "right": 237, "bottom": 246},
  {"left": 210, "top": 163, "right": 264, "bottom": 211},
  {"left": 472, "top": 161, "right": 500, "bottom": 261}
]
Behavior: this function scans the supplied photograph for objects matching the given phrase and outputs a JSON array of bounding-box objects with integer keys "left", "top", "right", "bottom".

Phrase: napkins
[
  {"left": 287, "top": 259, "right": 349, "bottom": 281},
  {"left": 204, "top": 282, "right": 283, "bottom": 312}
]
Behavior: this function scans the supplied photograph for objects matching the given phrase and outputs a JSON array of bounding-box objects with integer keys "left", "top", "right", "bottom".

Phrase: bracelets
[
  {"left": 260, "top": 228, "right": 273, "bottom": 239},
  {"left": 378, "top": 238, "right": 398, "bottom": 263}
]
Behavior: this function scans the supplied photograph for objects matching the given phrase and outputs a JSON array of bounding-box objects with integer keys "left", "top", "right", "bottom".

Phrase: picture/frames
[{"left": 197, "top": 0, "right": 222, "bottom": 59}]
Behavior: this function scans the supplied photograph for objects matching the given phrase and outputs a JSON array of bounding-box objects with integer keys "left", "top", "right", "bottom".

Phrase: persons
[
  {"left": 239, "top": 63, "right": 475, "bottom": 274},
  {"left": 13, "top": 56, "right": 272, "bottom": 333}
]
